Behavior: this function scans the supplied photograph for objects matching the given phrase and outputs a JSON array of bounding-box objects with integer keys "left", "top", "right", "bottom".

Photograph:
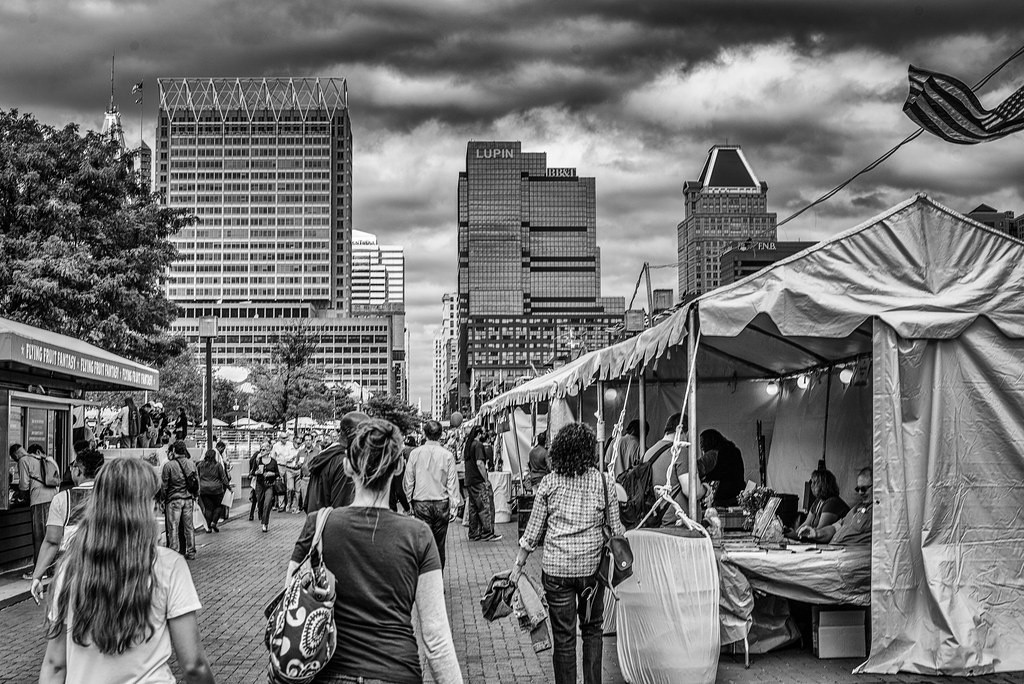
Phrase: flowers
[{"left": 736, "top": 484, "right": 778, "bottom": 529}]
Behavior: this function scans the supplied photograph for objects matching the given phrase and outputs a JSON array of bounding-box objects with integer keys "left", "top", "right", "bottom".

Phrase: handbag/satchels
[
  {"left": 185, "top": 474, "right": 199, "bottom": 495},
  {"left": 595, "top": 524, "right": 634, "bottom": 589},
  {"left": 264, "top": 506, "right": 337, "bottom": 684}
]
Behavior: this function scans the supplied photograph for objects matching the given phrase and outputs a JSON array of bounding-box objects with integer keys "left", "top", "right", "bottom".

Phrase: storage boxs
[{"left": 818, "top": 609, "right": 867, "bottom": 660}]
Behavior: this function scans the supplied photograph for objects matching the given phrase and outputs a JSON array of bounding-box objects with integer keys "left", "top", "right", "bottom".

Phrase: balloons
[{"left": 450, "top": 412, "right": 463, "bottom": 426}]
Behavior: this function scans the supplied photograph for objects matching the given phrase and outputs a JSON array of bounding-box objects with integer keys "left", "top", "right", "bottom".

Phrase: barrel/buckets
[{"left": 517, "top": 495, "right": 549, "bottom": 547}]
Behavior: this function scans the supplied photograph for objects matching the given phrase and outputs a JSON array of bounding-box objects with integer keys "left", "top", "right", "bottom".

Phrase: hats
[
  {"left": 154, "top": 402, "right": 163, "bottom": 408},
  {"left": 142, "top": 403, "right": 152, "bottom": 409}
]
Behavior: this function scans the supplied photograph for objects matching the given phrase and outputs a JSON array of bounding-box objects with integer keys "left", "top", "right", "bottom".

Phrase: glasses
[
  {"left": 855, "top": 484, "right": 873, "bottom": 493},
  {"left": 260, "top": 449, "right": 266, "bottom": 451},
  {"left": 282, "top": 436, "right": 288, "bottom": 439},
  {"left": 297, "top": 442, "right": 302, "bottom": 445}
]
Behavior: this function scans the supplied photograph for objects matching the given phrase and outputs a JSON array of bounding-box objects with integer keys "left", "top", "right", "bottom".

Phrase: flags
[{"left": 904, "top": 65, "right": 1024, "bottom": 145}]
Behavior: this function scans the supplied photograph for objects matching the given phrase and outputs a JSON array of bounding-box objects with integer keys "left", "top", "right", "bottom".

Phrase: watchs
[{"left": 515, "top": 557, "right": 526, "bottom": 566}]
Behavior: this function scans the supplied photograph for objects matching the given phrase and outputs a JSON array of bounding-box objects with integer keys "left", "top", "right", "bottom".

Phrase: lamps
[
  {"left": 604, "top": 384, "right": 617, "bottom": 400},
  {"left": 767, "top": 381, "right": 779, "bottom": 395},
  {"left": 797, "top": 373, "right": 811, "bottom": 389},
  {"left": 840, "top": 364, "right": 854, "bottom": 383}
]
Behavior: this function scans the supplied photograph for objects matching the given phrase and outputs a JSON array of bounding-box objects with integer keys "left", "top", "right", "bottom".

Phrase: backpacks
[
  {"left": 18, "top": 454, "right": 61, "bottom": 487},
  {"left": 616, "top": 442, "right": 681, "bottom": 531}
]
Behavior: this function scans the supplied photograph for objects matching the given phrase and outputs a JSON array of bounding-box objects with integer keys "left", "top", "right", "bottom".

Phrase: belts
[
  {"left": 277, "top": 463, "right": 286, "bottom": 467},
  {"left": 286, "top": 466, "right": 301, "bottom": 471}
]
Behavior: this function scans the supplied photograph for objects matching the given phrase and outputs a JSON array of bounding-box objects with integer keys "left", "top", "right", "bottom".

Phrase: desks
[{"left": 719, "top": 544, "right": 870, "bottom": 670}]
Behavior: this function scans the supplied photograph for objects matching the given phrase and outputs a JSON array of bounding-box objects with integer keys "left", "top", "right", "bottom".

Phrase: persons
[
  {"left": 604, "top": 421, "right": 650, "bottom": 477},
  {"left": 31, "top": 449, "right": 105, "bottom": 605},
  {"left": 250, "top": 411, "right": 503, "bottom": 581},
  {"left": 10, "top": 440, "right": 91, "bottom": 579},
  {"left": 284, "top": 420, "right": 463, "bottom": 684},
  {"left": 39, "top": 457, "right": 215, "bottom": 684},
  {"left": 697, "top": 429, "right": 747, "bottom": 507},
  {"left": 195, "top": 450, "right": 232, "bottom": 533},
  {"left": 641, "top": 412, "right": 712, "bottom": 526},
  {"left": 529, "top": 432, "right": 552, "bottom": 495},
  {"left": 798, "top": 468, "right": 873, "bottom": 648},
  {"left": 213, "top": 442, "right": 235, "bottom": 523},
  {"left": 783, "top": 469, "right": 850, "bottom": 539},
  {"left": 86, "top": 395, "right": 188, "bottom": 448},
  {"left": 157, "top": 440, "right": 200, "bottom": 559},
  {"left": 508, "top": 422, "right": 622, "bottom": 684}
]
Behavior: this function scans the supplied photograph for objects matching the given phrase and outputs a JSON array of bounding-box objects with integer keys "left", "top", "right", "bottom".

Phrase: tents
[{"left": 458, "top": 195, "right": 1024, "bottom": 684}]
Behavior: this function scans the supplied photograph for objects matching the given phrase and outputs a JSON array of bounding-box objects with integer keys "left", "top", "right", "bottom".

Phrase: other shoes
[
  {"left": 249, "top": 516, "right": 253, "bottom": 521},
  {"left": 211, "top": 523, "right": 220, "bottom": 533},
  {"left": 185, "top": 553, "right": 195, "bottom": 560},
  {"left": 469, "top": 536, "right": 481, "bottom": 541},
  {"left": 206, "top": 530, "right": 211, "bottom": 533},
  {"left": 489, "top": 534, "right": 502, "bottom": 541},
  {"left": 262, "top": 528, "right": 268, "bottom": 533},
  {"left": 23, "top": 572, "right": 47, "bottom": 579}
]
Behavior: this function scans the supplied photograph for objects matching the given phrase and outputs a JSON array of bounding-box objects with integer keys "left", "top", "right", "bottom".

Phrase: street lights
[{"left": 197, "top": 316, "right": 219, "bottom": 451}]
